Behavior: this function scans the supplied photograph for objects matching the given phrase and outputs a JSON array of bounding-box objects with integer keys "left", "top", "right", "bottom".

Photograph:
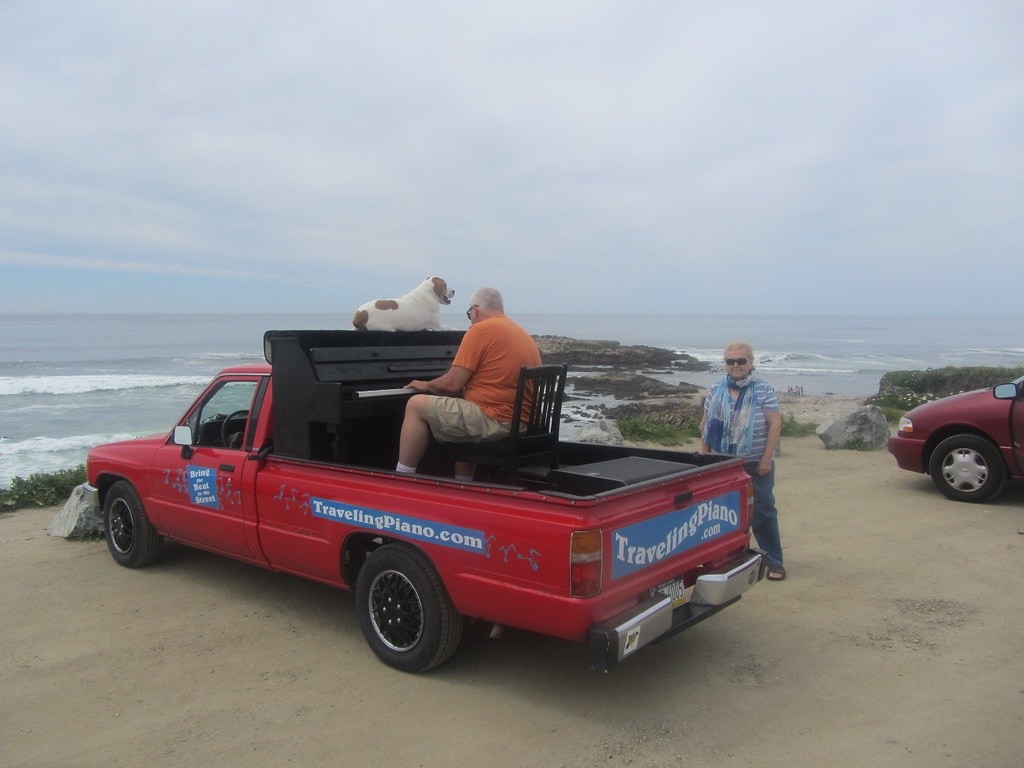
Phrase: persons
[
  {"left": 699, "top": 344, "right": 786, "bottom": 582},
  {"left": 394, "top": 287, "right": 543, "bottom": 473}
]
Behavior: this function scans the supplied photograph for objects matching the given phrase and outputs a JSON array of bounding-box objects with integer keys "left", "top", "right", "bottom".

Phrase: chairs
[{"left": 445, "top": 363, "right": 568, "bottom": 485}]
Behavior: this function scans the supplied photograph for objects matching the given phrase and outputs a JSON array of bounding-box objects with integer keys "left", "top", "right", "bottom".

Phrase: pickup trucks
[{"left": 81, "top": 329, "right": 769, "bottom": 675}]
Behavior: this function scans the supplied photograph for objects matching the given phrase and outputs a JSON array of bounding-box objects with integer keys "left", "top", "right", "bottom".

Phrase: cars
[{"left": 886, "top": 374, "right": 1024, "bottom": 504}]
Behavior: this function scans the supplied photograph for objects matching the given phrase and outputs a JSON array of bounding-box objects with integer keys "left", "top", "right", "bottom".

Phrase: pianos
[{"left": 262, "top": 329, "right": 467, "bottom": 463}]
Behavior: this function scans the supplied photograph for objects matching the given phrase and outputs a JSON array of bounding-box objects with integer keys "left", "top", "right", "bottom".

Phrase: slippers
[{"left": 766, "top": 566, "right": 786, "bottom": 581}]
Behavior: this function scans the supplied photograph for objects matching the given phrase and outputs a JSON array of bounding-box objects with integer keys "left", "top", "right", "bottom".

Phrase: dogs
[{"left": 351, "top": 276, "right": 459, "bottom": 331}]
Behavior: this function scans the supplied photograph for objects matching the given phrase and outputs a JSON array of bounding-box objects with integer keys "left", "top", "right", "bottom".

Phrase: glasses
[
  {"left": 466, "top": 305, "right": 479, "bottom": 320},
  {"left": 726, "top": 358, "right": 750, "bottom": 366}
]
